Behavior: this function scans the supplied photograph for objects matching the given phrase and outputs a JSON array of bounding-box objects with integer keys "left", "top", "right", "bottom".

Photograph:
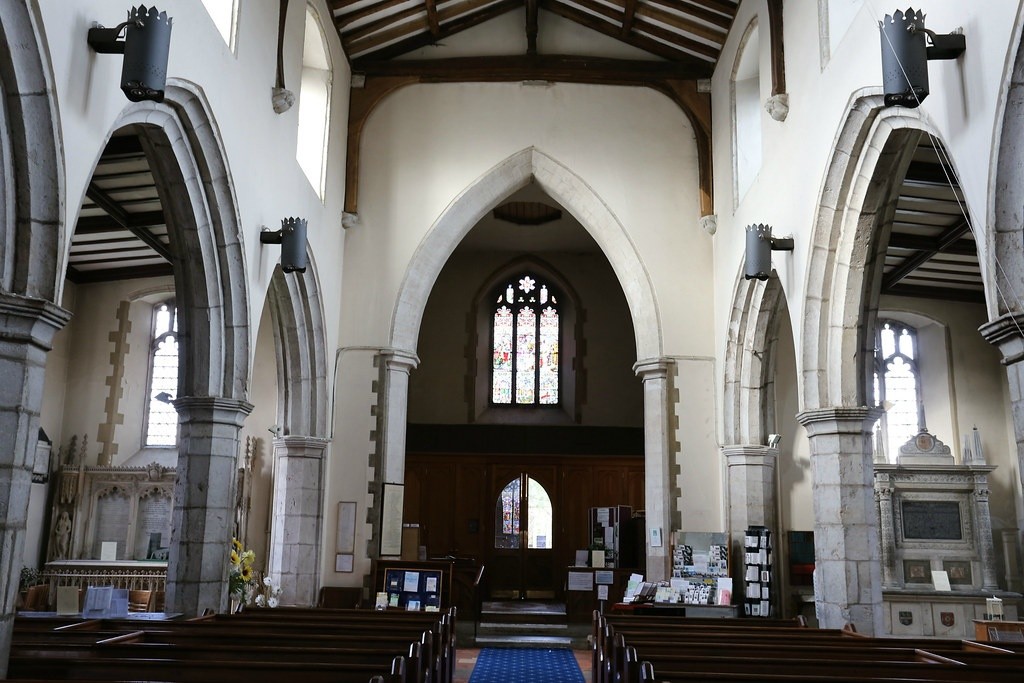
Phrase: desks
[
  {"left": 566, "top": 566, "right": 645, "bottom": 611},
  {"left": 972, "top": 619, "right": 1024, "bottom": 643},
  {"left": 372, "top": 560, "right": 454, "bottom": 607}
]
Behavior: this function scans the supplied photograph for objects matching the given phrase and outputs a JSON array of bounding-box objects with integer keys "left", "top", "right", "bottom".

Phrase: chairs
[
  {"left": 453, "top": 584, "right": 488, "bottom": 637},
  {"left": 317, "top": 586, "right": 364, "bottom": 609}
]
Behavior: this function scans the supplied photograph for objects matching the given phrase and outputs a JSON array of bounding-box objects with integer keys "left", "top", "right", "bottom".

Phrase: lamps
[
  {"left": 768, "top": 433, "right": 782, "bottom": 448},
  {"left": 260, "top": 216, "right": 308, "bottom": 273},
  {"left": 877, "top": 8, "right": 966, "bottom": 107},
  {"left": 744, "top": 223, "right": 794, "bottom": 281},
  {"left": 87, "top": 4, "right": 173, "bottom": 103}
]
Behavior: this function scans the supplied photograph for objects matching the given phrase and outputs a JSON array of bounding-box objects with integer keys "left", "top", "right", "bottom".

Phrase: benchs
[
  {"left": 5, "top": 606, "right": 456, "bottom": 683},
  {"left": 588, "top": 610, "right": 1024, "bottom": 683}
]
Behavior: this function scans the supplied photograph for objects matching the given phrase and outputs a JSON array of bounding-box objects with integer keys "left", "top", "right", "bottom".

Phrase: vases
[{"left": 230, "top": 564, "right": 242, "bottom": 613}]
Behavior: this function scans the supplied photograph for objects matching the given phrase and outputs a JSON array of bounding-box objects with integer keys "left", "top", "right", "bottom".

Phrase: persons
[{"left": 55, "top": 512, "right": 71, "bottom": 559}]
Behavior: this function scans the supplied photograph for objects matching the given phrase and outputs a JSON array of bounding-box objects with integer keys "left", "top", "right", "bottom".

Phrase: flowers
[{"left": 229, "top": 537, "right": 256, "bottom": 593}]
